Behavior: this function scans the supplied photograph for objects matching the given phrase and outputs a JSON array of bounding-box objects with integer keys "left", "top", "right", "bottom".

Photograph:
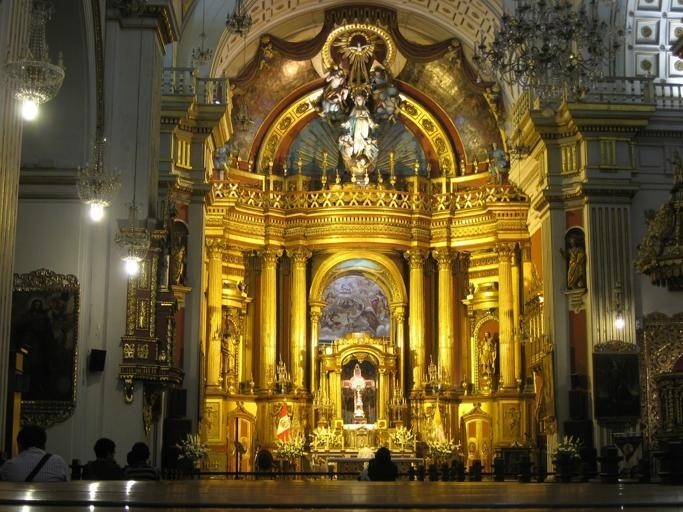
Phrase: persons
[
  {"left": 124, "top": 442, "right": 154, "bottom": 478},
  {"left": 21, "top": 300, "right": 57, "bottom": 388},
  {"left": 477, "top": 331, "right": 496, "bottom": 373},
  {"left": 0, "top": 425, "right": 67, "bottom": 481},
  {"left": 323, "top": 63, "right": 395, "bottom": 152},
  {"left": 565, "top": 240, "right": 585, "bottom": 286},
  {"left": 356, "top": 447, "right": 390, "bottom": 479},
  {"left": 86, "top": 437, "right": 119, "bottom": 478},
  {"left": 217, "top": 315, "right": 235, "bottom": 373}
]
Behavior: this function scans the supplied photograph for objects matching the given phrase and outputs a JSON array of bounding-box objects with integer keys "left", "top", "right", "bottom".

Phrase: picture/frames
[
  {"left": 592, "top": 339, "right": 645, "bottom": 429},
  {"left": 7, "top": 268, "right": 80, "bottom": 432}
]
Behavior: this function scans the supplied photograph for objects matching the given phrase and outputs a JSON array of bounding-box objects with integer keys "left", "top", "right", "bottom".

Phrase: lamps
[
  {"left": 0, "top": 1, "right": 63, "bottom": 123},
  {"left": 610, "top": 281, "right": 625, "bottom": 332},
  {"left": 76, "top": 0, "right": 126, "bottom": 222},
  {"left": 113, "top": 1, "right": 152, "bottom": 276},
  {"left": 470, "top": 0, "right": 625, "bottom": 109}
]
